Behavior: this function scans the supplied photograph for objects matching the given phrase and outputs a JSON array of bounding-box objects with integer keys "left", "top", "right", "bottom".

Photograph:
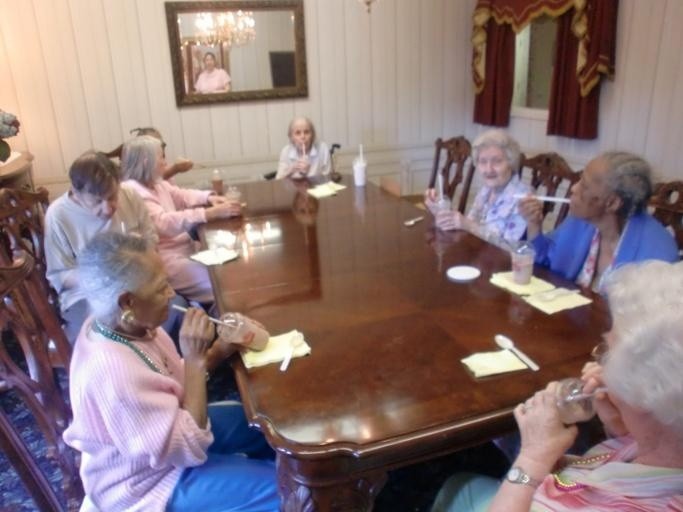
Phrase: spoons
[
  {"left": 494, "top": 333, "right": 539, "bottom": 372},
  {"left": 279, "top": 333, "right": 303, "bottom": 370},
  {"left": 404, "top": 216, "right": 423, "bottom": 226}
]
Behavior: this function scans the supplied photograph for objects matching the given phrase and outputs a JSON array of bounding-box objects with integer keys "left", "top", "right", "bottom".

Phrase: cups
[
  {"left": 558, "top": 378, "right": 597, "bottom": 424},
  {"left": 432, "top": 196, "right": 452, "bottom": 213},
  {"left": 298, "top": 154, "right": 310, "bottom": 174},
  {"left": 353, "top": 158, "right": 368, "bottom": 187},
  {"left": 225, "top": 187, "right": 243, "bottom": 218},
  {"left": 511, "top": 240, "right": 535, "bottom": 285},
  {"left": 215, "top": 313, "right": 269, "bottom": 352}
]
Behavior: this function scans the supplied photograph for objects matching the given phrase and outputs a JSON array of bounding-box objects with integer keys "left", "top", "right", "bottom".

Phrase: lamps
[{"left": 195, "top": 10, "right": 255, "bottom": 48}]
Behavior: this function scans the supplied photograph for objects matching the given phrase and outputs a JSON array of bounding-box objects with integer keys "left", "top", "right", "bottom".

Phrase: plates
[{"left": 446, "top": 266, "right": 482, "bottom": 282}]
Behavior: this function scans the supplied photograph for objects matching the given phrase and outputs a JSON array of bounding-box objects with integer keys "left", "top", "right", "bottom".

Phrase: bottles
[{"left": 208, "top": 169, "right": 223, "bottom": 195}]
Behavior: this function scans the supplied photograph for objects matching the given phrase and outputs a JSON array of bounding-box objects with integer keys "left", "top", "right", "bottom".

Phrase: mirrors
[{"left": 164, "top": 1, "right": 308, "bottom": 107}]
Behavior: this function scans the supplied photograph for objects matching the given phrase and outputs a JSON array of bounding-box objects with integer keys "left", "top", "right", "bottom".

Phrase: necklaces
[
  {"left": 550, "top": 450, "right": 615, "bottom": 491},
  {"left": 91, "top": 320, "right": 173, "bottom": 378}
]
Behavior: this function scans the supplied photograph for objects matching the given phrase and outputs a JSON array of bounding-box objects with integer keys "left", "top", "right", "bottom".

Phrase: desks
[{"left": 0, "top": 151, "right": 44, "bottom": 221}]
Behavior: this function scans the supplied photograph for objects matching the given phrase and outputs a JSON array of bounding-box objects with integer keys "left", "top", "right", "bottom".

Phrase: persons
[
  {"left": 62, "top": 231, "right": 281, "bottom": 512},
  {"left": 511, "top": 150, "right": 678, "bottom": 310},
  {"left": 422, "top": 129, "right": 543, "bottom": 251},
  {"left": 136, "top": 128, "right": 192, "bottom": 179},
  {"left": 427, "top": 261, "right": 683, "bottom": 512},
  {"left": 286, "top": 180, "right": 323, "bottom": 224},
  {"left": 44, "top": 153, "right": 186, "bottom": 341},
  {"left": 192, "top": 51, "right": 234, "bottom": 94},
  {"left": 117, "top": 139, "right": 243, "bottom": 314},
  {"left": 273, "top": 117, "right": 332, "bottom": 180}
]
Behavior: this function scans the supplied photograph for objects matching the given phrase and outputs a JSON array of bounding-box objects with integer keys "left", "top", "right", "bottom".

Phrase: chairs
[
  {"left": 401, "top": 135, "right": 475, "bottom": 215},
  {"left": 645, "top": 180, "right": 683, "bottom": 250},
  {"left": 519, "top": 152, "right": 583, "bottom": 229},
  {"left": 0, "top": 184, "right": 85, "bottom": 512}
]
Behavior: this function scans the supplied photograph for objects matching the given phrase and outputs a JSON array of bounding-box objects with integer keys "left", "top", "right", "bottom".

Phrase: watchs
[{"left": 498, "top": 466, "right": 542, "bottom": 490}]
